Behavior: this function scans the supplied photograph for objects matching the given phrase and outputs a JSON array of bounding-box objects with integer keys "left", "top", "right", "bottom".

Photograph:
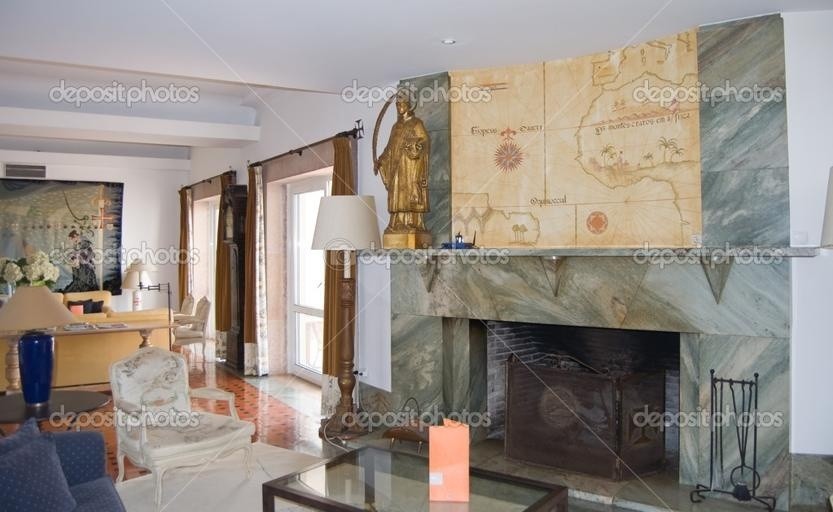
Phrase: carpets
[{"left": 115, "top": 441, "right": 527, "bottom": 511}]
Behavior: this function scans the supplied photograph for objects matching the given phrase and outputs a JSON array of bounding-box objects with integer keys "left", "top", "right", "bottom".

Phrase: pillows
[
  {"left": 68, "top": 299, "right": 104, "bottom": 315},
  {"left": 0, "top": 431, "right": 76, "bottom": 511},
  {"left": 0, "top": 417, "right": 40, "bottom": 456}
]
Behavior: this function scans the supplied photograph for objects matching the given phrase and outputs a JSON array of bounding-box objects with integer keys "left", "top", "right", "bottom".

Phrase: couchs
[{"left": 52, "top": 290, "right": 114, "bottom": 320}]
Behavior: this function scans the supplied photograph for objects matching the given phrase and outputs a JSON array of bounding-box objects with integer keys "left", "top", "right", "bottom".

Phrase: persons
[{"left": 370, "top": 89, "right": 430, "bottom": 234}]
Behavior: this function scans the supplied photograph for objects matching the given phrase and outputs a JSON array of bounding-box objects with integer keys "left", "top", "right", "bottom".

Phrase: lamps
[
  {"left": 1, "top": 285, "right": 74, "bottom": 408},
  {"left": 311, "top": 195, "right": 383, "bottom": 440},
  {"left": 120, "top": 258, "right": 152, "bottom": 312}
]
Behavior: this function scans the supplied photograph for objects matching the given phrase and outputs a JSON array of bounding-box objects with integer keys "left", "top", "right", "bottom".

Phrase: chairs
[
  {"left": 0, "top": 418, "right": 126, "bottom": 511},
  {"left": 173, "top": 294, "right": 211, "bottom": 370},
  {"left": 108, "top": 348, "right": 255, "bottom": 506}
]
